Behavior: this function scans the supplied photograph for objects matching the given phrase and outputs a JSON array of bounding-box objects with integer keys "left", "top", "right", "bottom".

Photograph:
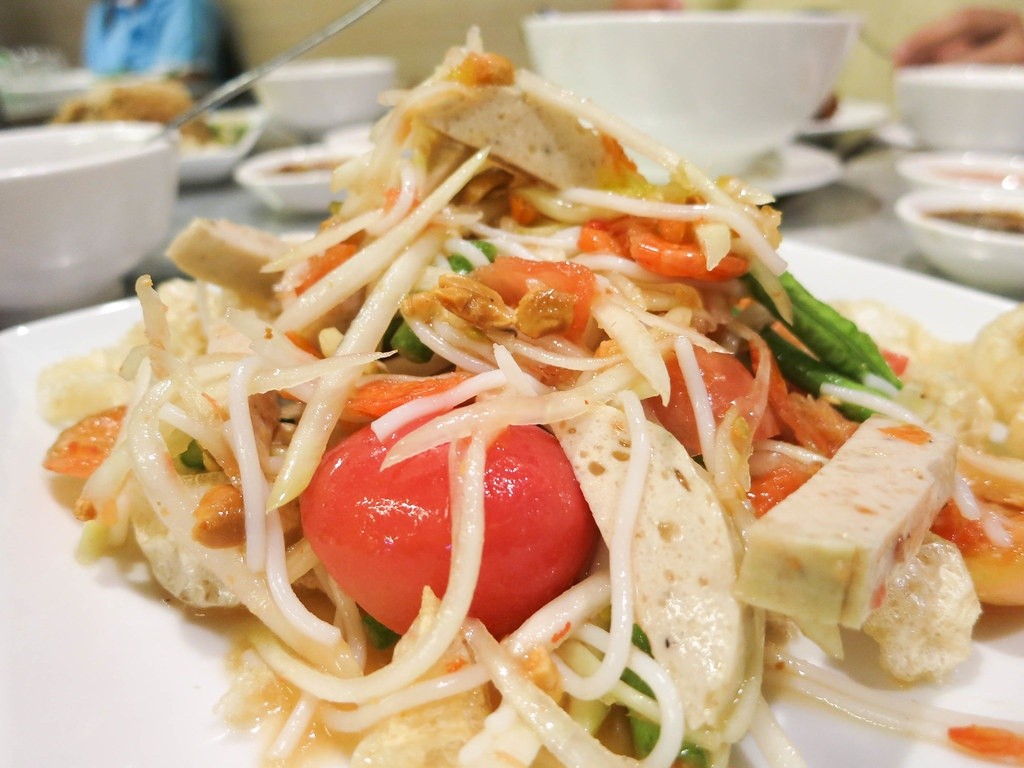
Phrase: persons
[
  {"left": 79, "top": 0, "right": 228, "bottom": 106},
  {"left": 888, "top": 7, "right": 1023, "bottom": 68}
]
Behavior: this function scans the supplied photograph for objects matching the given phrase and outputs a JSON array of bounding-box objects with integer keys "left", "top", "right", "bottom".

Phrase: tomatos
[{"left": 42, "top": 183, "right": 983, "bottom": 646}]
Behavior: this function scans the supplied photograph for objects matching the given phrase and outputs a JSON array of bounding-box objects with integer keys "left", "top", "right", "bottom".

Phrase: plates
[
  {"left": 896, "top": 151, "right": 1024, "bottom": 188},
  {"left": 799, "top": 98, "right": 892, "bottom": 149},
  {"left": 175, "top": 112, "right": 267, "bottom": 192},
  {"left": 1, "top": 232, "right": 1024, "bottom": 767},
  {"left": 739, "top": 148, "right": 844, "bottom": 212},
  {"left": 233, "top": 146, "right": 369, "bottom": 216}
]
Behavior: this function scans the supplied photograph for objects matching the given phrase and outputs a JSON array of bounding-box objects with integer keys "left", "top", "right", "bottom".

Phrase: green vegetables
[{"left": 208, "top": 124, "right": 248, "bottom": 146}]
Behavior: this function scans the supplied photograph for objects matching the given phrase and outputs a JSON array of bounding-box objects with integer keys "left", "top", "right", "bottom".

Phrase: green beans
[
  {"left": 392, "top": 239, "right": 496, "bottom": 362},
  {"left": 180, "top": 430, "right": 207, "bottom": 468},
  {"left": 733, "top": 310, "right": 894, "bottom": 421},
  {"left": 742, "top": 255, "right": 900, "bottom": 389},
  {"left": 623, "top": 618, "right": 708, "bottom": 765}
]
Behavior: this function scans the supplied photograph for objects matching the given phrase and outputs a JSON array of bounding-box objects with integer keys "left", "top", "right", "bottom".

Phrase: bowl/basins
[
  {"left": 516, "top": 12, "right": 859, "bottom": 187},
  {"left": 888, "top": 64, "right": 1024, "bottom": 160},
  {"left": 248, "top": 55, "right": 396, "bottom": 135},
  {"left": 1, "top": 117, "right": 177, "bottom": 318},
  {"left": 893, "top": 185, "right": 1024, "bottom": 297}
]
